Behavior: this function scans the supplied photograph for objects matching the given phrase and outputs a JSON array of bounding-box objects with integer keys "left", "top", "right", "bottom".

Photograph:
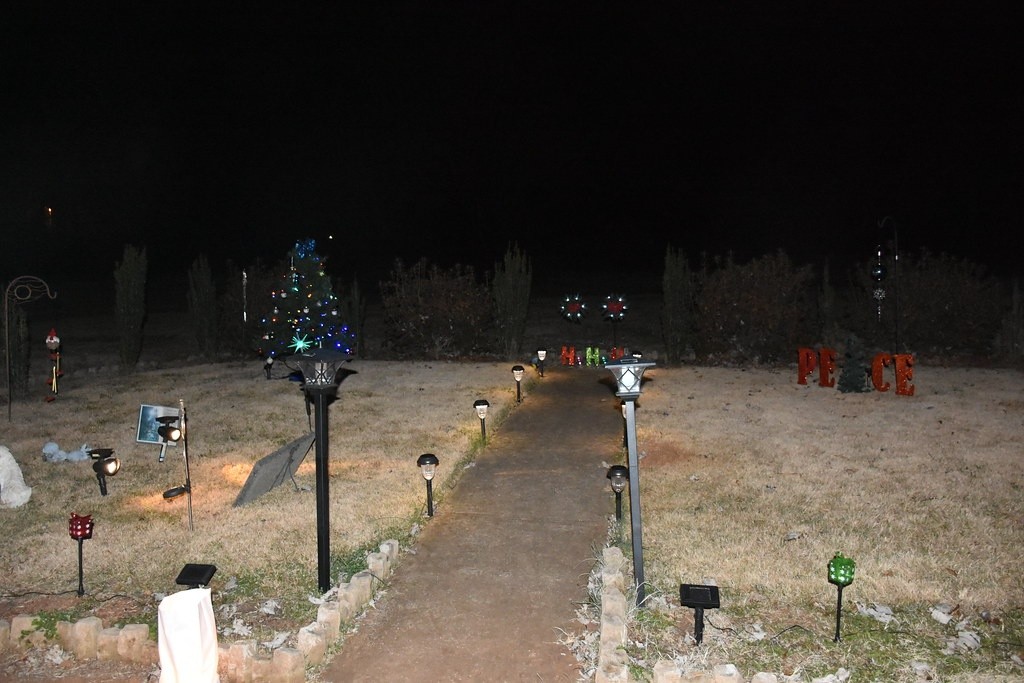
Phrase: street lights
[
  {"left": 286, "top": 341, "right": 355, "bottom": 594},
  {"left": 605, "top": 361, "right": 658, "bottom": 609}
]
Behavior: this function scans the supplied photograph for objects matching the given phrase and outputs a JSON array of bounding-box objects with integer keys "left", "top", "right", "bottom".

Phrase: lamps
[
  {"left": 282, "top": 335, "right": 657, "bottom": 614},
  {"left": 85, "top": 448, "right": 122, "bottom": 496},
  {"left": 155, "top": 415, "right": 181, "bottom": 462}
]
